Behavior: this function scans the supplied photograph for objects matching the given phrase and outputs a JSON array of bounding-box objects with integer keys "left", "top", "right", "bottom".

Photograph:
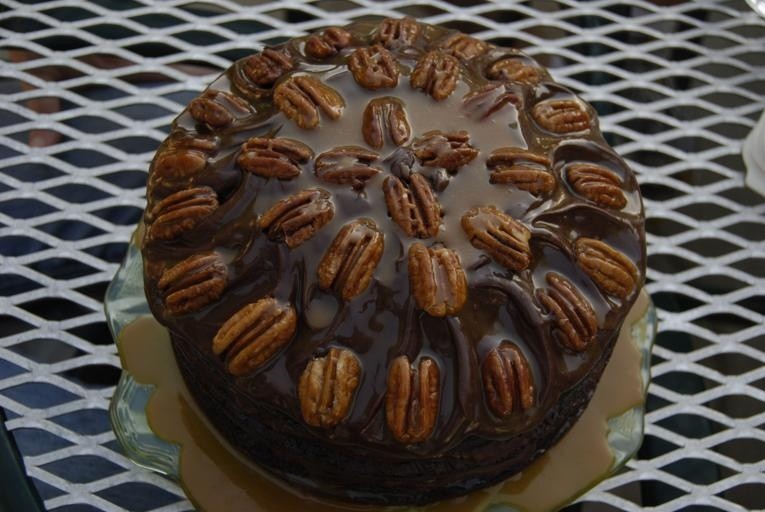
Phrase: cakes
[{"left": 144, "top": 13, "right": 639, "bottom": 512}]
